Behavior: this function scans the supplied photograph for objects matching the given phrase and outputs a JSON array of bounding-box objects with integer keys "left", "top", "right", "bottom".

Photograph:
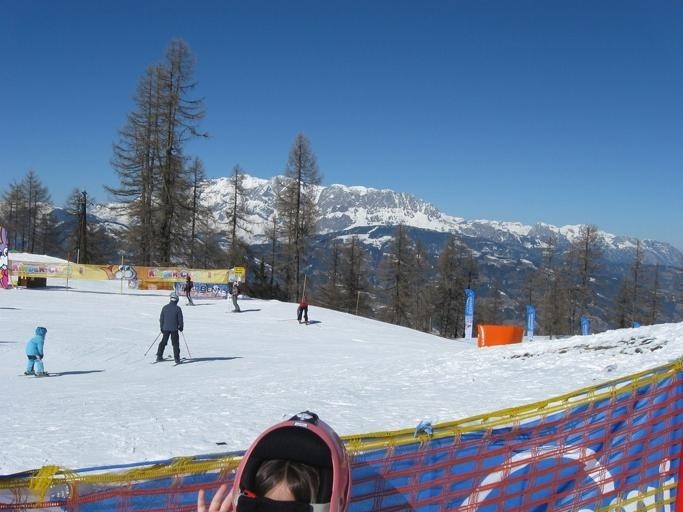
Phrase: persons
[
  {"left": 296, "top": 295, "right": 309, "bottom": 324},
  {"left": 183, "top": 276, "right": 194, "bottom": 306},
  {"left": 196, "top": 412, "right": 352, "bottom": 510},
  {"left": 22, "top": 327, "right": 49, "bottom": 377},
  {"left": 154, "top": 292, "right": 185, "bottom": 363},
  {"left": 230, "top": 281, "right": 241, "bottom": 312}
]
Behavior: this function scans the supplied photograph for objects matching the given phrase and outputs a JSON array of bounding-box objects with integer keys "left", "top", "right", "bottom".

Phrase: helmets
[
  {"left": 229, "top": 411, "right": 351, "bottom": 512},
  {"left": 170, "top": 294, "right": 179, "bottom": 302}
]
